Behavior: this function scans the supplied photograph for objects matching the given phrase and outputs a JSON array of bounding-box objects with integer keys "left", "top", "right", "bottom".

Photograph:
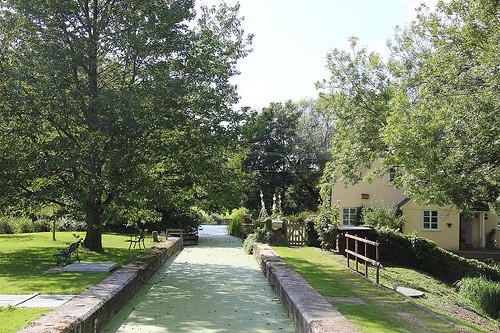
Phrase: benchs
[
  {"left": 53, "top": 239, "right": 82, "bottom": 267},
  {"left": 126, "top": 228, "right": 147, "bottom": 250}
]
